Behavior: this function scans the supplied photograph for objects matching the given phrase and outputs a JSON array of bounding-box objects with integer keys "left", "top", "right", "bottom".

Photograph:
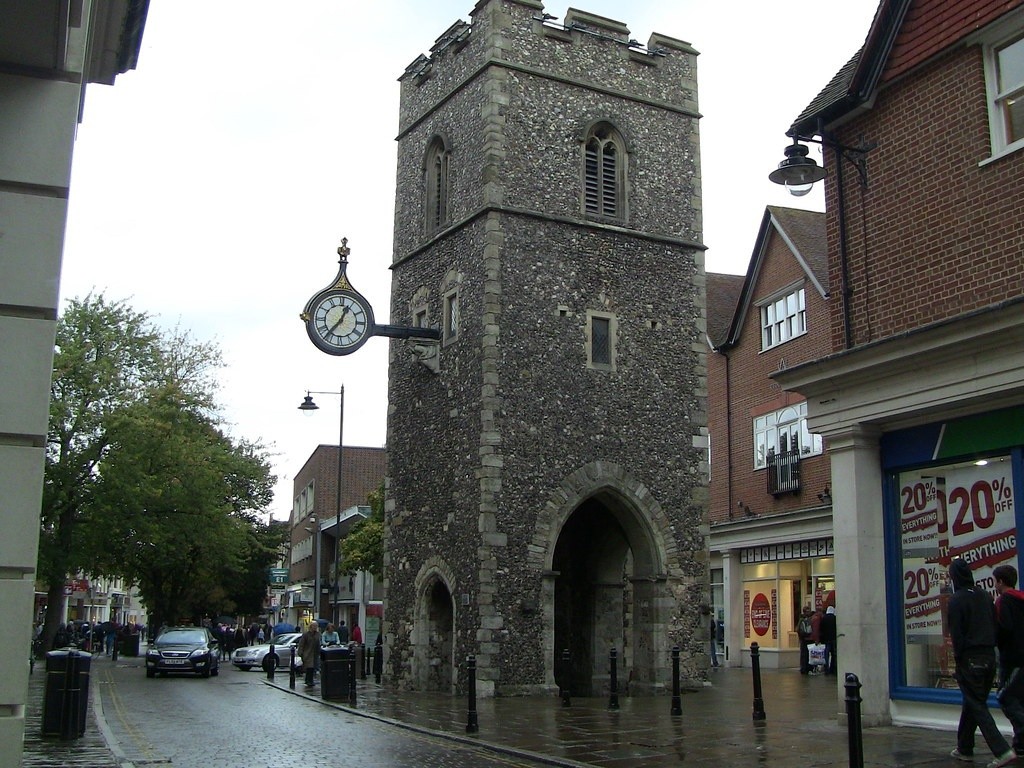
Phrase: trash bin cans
[
  {"left": 346, "top": 642, "right": 366, "bottom": 679},
  {"left": 41, "top": 650, "right": 92, "bottom": 739},
  {"left": 60, "top": 647, "right": 77, "bottom": 651},
  {"left": 320, "top": 642, "right": 352, "bottom": 701},
  {"left": 123, "top": 634, "right": 140, "bottom": 656},
  {"left": 67, "top": 643, "right": 76, "bottom": 647}
]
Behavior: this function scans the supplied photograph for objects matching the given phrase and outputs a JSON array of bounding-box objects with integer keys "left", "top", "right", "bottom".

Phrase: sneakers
[
  {"left": 986, "top": 747, "right": 1017, "bottom": 768},
  {"left": 950, "top": 750, "right": 974, "bottom": 760}
]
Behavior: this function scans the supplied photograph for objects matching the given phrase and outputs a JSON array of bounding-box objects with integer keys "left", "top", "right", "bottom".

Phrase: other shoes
[
  {"left": 1016, "top": 751, "right": 1024, "bottom": 757},
  {"left": 808, "top": 671, "right": 816, "bottom": 676},
  {"left": 308, "top": 682, "right": 315, "bottom": 686},
  {"left": 713, "top": 665, "right": 722, "bottom": 668}
]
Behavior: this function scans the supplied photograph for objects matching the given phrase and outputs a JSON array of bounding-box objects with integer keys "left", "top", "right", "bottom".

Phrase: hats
[
  {"left": 710, "top": 611, "right": 714, "bottom": 615},
  {"left": 826, "top": 606, "right": 835, "bottom": 614}
]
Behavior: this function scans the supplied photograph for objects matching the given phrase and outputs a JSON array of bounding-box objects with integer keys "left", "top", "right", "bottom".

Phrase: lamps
[
  {"left": 569, "top": 23, "right": 587, "bottom": 32},
  {"left": 654, "top": 47, "right": 672, "bottom": 54},
  {"left": 738, "top": 501, "right": 752, "bottom": 517},
  {"left": 414, "top": 59, "right": 431, "bottom": 67},
  {"left": 769, "top": 131, "right": 871, "bottom": 197},
  {"left": 824, "top": 479, "right": 833, "bottom": 494},
  {"left": 630, "top": 39, "right": 644, "bottom": 46},
  {"left": 310, "top": 513, "right": 322, "bottom": 524},
  {"left": 442, "top": 35, "right": 459, "bottom": 45},
  {"left": 407, "top": 70, "right": 421, "bottom": 75},
  {"left": 540, "top": 13, "right": 558, "bottom": 23},
  {"left": 430, "top": 49, "right": 444, "bottom": 57}
]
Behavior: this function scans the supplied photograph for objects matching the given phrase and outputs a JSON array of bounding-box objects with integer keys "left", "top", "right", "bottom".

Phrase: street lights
[
  {"left": 296, "top": 382, "right": 346, "bottom": 625},
  {"left": 309, "top": 512, "right": 322, "bottom": 623}
]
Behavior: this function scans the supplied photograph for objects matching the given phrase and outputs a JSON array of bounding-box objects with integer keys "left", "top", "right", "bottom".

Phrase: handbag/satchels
[
  {"left": 806, "top": 642, "right": 825, "bottom": 665},
  {"left": 323, "top": 640, "right": 337, "bottom": 648}
]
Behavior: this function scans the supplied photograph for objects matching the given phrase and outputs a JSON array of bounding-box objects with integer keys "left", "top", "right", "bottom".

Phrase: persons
[
  {"left": 336, "top": 621, "right": 349, "bottom": 643},
  {"left": 711, "top": 618, "right": 722, "bottom": 667},
  {"left": 298, "top": 621, "right": 321, "bottom": 686},
  {"left": 322, "top": 623, "right": 340, "bottom": 646},
  {"left": 33, "top": 618, "right": 149, "bottom": 656},
  {"left": 797, "top": 606, "right": 836, "bottom": 677},
  {"left": 160, "top": 617, "right": 301, "bottom": 662},
  {"left": 992, "top": 565, "right": 1024, "bottom": 758},
  {"left": 947, "top": 558, "right": 1017, "bottom": 768},
  {"left": 352, "top": 622, "right": 363, "bottom": 645}
]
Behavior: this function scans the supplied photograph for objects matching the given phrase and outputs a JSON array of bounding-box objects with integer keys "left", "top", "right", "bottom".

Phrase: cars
[
  {"left": 231, "top": 632, "right": 302, "bottom": 672},
  {"left": 146, "top": 626, "right": 223, "bottom": 679}
]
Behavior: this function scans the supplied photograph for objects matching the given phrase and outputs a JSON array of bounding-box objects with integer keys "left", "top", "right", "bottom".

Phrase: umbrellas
[
  {"left": 273, "top": 622, "right": 295, "bottom": 634},
  {"left": 316, "top": 618, "right": 331, "bottom": 627}
]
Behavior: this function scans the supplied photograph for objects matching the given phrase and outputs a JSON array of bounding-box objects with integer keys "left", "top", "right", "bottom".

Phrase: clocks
[{"left": 315, "top": 291, "right": 365, "bottom": 347}]
